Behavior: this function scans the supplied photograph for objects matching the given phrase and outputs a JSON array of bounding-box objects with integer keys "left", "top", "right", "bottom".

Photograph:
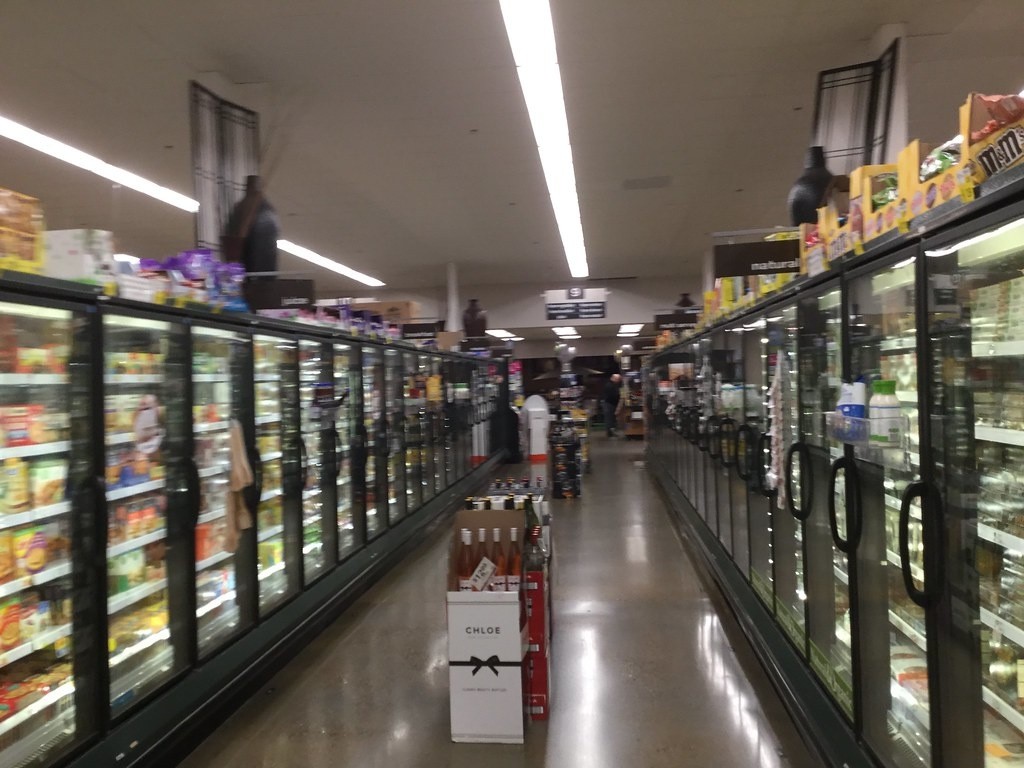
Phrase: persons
[{"left": 601, "top": 374, "right": 621, "bottom": 438}]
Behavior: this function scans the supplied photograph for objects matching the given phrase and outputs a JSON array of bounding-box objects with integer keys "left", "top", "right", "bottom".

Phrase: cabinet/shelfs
[{"left": 532, "top": 372, "right": 594, "bottom": 477}]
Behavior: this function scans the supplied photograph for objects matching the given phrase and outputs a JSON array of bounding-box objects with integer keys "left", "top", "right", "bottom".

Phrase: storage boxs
[
  {"left": 44, "top": 228, "right": 114, "bottom": 279},
  {"left": 445, "top": 507, "right": 549, "bottom": 744},
  {"left": 0, "top": 186, "right": 40, "bottom": 262},
  {"left": 246, "top": 277, "right": 317, "bottom": 310},
  {"left": 436, "top": 330, "right": 462, "bottom": 351},
  {"left": 356, "top": 301, "right": 419, "bottom": 325}
]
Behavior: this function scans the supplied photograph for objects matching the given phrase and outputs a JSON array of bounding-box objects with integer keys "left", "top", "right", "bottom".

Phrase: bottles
[
  {"left": 456, "top": 527, "right": 472, "bottom": 592},
  {"left": 490, "top": 528, "right": 506, "bottom": 591},
  {"left": 463, "top": 475, "right": 552, "bottom": 570},
  {"left": 787, "top": 147, "right": 834, "bottom": 227},
  {"left": 462, "top": 299, "right": 487, "bottom": 347},
  {"left": 506, "top": 528, "right": 522, "bottom": 592},
  {"left": 222, "top": 172, "right": 277, "bottom": 281},
  {"left": 832, "top": 376, "right": 901, "bottom": 448},
  {"left": 473, "top": 528, "right": 490, "bottom": 591}
]
